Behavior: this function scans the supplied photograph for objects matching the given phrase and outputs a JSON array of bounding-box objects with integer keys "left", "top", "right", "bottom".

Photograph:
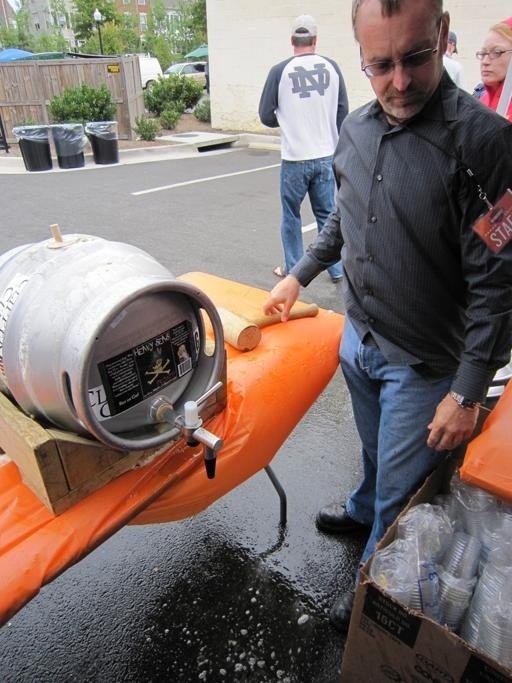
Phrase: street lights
[{"left": 94, "top": 8, "right": 104, "bottom": 55}]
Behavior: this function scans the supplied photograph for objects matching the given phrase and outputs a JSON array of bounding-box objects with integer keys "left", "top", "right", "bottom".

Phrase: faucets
[{"left": 149, "top": 381, "right": 223, "bottom": 479}]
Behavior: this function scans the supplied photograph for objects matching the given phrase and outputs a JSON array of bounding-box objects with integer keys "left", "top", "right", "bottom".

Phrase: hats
[
  {"left": 448, "top": 31, "right": 457, "bottom": 54},
  {"left": 291, "top": 15, "right": 318, "bottom": 37}
]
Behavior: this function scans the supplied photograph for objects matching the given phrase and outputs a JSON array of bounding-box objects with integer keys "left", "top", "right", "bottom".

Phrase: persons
[
  {"left": 443, "top": 31, "right": 464, "bottom": 88},
  {"left": 470, "top": 16, "right": 512, "bottom": 123},
  {"left": 261, "top": 0, "right": 512, "bottom": 637},
  {"left": 259, "top": 14, "right": 343, "bottom": 284}
]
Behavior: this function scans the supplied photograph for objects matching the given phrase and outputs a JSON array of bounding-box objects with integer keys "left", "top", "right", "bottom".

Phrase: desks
[
  {"left": 460, "top": 375, "right": 512, "bottom": 501},
  {"left": 1, "top": 271, "right": 346, "bottom": 631}
]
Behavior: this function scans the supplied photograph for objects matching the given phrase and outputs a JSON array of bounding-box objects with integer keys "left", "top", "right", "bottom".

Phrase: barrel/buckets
[{"left": 0, "top": 223, "right": 225, "bottom": 452}]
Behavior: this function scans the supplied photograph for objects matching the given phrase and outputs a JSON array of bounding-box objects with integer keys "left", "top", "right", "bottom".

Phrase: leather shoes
[{"left": 316, "top": 500, "right": 355, "bottom": 631}]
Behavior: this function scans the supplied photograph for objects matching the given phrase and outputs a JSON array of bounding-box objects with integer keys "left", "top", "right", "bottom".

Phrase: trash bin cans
[
  {"left": 49, "top": 122, "right": 86, "bottom": 170},
  {"left": 10, "top": 124, "right": 53, "bottom": 172},
  {"left": 85, "top": 121, "right": 120, "bottom": 165}
]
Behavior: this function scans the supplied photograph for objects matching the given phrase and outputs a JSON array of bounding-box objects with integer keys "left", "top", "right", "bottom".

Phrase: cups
[{"left": 367, "top": 465, "right": 511, "bottom": 664}]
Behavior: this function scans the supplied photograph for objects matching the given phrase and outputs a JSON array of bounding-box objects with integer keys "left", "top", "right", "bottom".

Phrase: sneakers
[{"left": 272, "top": 265, "right": 285, "bottom": 277}]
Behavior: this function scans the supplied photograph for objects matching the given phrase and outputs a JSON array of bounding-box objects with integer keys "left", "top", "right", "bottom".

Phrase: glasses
[
  {"left": 475, "top": 48, "right": 507, "bottom": 61},
  {"left": 359, "top": 15, "right": 442, "bottom": 78}
]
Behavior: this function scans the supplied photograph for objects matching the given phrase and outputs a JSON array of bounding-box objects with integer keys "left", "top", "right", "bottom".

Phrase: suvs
[
  {"left": 160, "top": 61, "right": 208, "bottom": 89},
  {"left": 138, "top": 52, "right": 163, "bottom": 91}
]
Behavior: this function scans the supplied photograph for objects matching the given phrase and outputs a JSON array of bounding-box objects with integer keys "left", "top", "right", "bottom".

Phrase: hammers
[{"left": 218, "top": 302, "right": 319, "bottom": 354}]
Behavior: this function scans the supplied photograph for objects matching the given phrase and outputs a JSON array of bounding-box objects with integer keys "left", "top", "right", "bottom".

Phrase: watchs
[{"left": 448, "top": 390, "right": 480, "bottom": 409}]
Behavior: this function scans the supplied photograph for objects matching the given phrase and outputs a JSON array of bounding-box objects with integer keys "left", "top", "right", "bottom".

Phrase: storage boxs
[{"left": 338, "top": 405, "right": 512, "bottom": 681}]
